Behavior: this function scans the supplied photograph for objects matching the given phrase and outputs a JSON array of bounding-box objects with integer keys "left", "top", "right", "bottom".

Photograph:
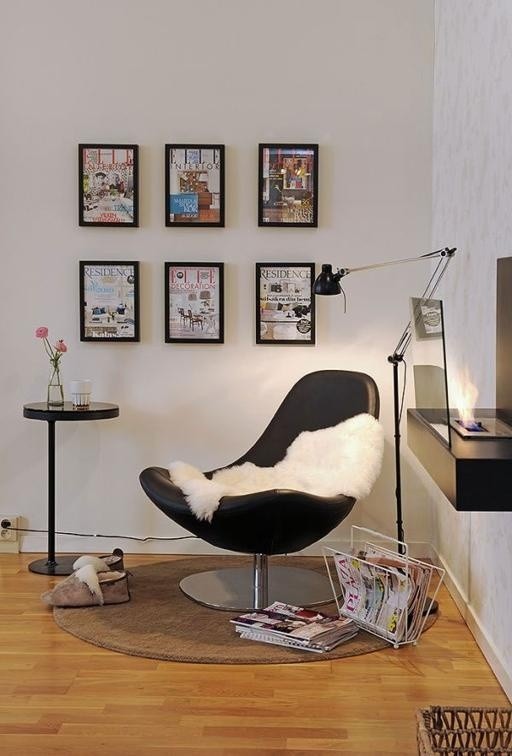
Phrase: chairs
[
  {"left": 414, "top": 364, "right": 451, "bottom": 445},
  {"left": 138, "top": 370, "right": 381, "bottom": 615}
]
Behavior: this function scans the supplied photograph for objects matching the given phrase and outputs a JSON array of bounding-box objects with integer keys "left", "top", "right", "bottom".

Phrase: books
[{"left": 229, "top": 545, "right": 432, "bottom": 654}]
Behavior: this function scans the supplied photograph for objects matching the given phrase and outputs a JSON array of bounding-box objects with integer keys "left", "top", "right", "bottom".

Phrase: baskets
[{"left": 416, "top": 705, "right": 512, "bottom": 756}]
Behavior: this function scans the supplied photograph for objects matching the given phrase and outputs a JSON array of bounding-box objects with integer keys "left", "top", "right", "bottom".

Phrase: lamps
[{"left": 314, "top": 246, "right": 460, "bottom": 621}]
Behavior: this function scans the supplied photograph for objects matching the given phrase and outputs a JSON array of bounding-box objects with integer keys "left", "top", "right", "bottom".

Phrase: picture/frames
[
  {"left": 164, "top": 261, "right": 224, "bottom": 344},
  {"left": 78, "top": 143, "right": 139, "bottom": 227},
  {"left": 256, "top": 263, "right": 315, "bottom": 343},
  {"left": 165, "top": 143, "right": 224, "bottom": 227},
  {"left": 258, "top": 143, "right": 317, "bottom": 226},
  {"left": 411, "top": 297, "right": 442, "bottom": 341},
  {"left": 443, "top": 416, "right": 512, "bottom": 438},
  {"left": 79, "top": 260, "right": 139, "bottom": 342}
]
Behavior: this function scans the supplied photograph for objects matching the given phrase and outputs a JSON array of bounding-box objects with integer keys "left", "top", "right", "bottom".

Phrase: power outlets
[{"left": 0, "top": 514, "right": 21, "bottom": 543}]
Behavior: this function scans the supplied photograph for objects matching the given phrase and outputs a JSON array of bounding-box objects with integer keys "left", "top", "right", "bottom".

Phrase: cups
[{"left": 69, "top": 377, "right": 90, "bottom": 408}]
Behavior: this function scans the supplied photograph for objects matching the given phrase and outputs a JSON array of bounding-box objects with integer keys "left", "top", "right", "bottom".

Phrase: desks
[
  {"left": 405, "top": 406, "right": 512, "bottom": 512},
  {"left": 23, "top": 400, "right": 120, "bottom": 575}
]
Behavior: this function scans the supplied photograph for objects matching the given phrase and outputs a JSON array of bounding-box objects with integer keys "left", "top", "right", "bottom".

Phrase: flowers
[{"left": 35, "top": 327, "right": 67, "bottom": 402}]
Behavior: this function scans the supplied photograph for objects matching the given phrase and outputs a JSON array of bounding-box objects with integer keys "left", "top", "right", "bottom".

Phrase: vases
[{"left": 47, "top": 361, "right": 65, "bottom": 406}]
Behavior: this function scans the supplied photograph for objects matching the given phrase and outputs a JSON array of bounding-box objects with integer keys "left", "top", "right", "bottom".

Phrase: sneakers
[
  {"left": 50, "top": 564, "right": 130, "bottom": 608},
  {"left": 41, "top": 549, "right": 123, "bottom": 601}
]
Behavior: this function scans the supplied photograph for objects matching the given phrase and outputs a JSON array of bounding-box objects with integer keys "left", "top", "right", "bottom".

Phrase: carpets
[{"left": 52, "top": 554, "right": 441, "bottom": 664}]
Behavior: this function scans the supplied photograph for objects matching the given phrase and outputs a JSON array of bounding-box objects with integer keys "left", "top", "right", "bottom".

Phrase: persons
[{"left": 95, "top": 171, "right": 123, "bottom": 191}]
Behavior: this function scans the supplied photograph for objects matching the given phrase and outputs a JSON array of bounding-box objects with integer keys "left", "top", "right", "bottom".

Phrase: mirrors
[{"left": 408, "top": 295, "right": 452, "bottom": 448}]
